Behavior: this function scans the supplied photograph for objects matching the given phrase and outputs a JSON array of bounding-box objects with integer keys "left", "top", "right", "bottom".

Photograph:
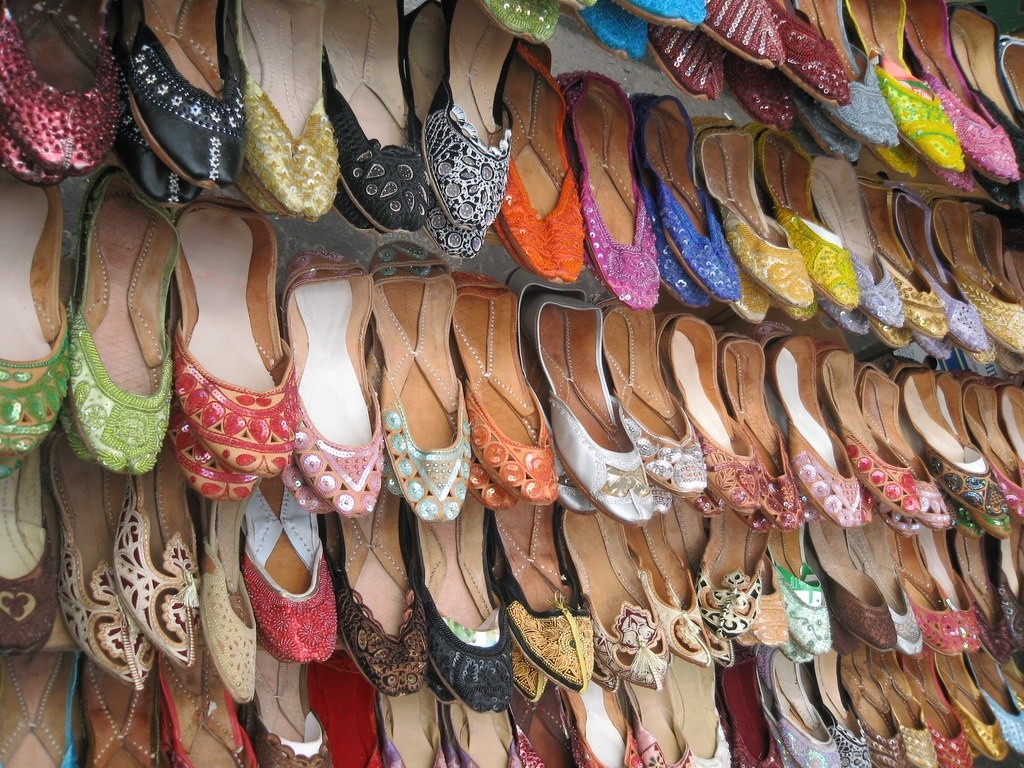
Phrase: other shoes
[{"left": 0, "top": 1, "right": 1024, "bottom": 768}]
